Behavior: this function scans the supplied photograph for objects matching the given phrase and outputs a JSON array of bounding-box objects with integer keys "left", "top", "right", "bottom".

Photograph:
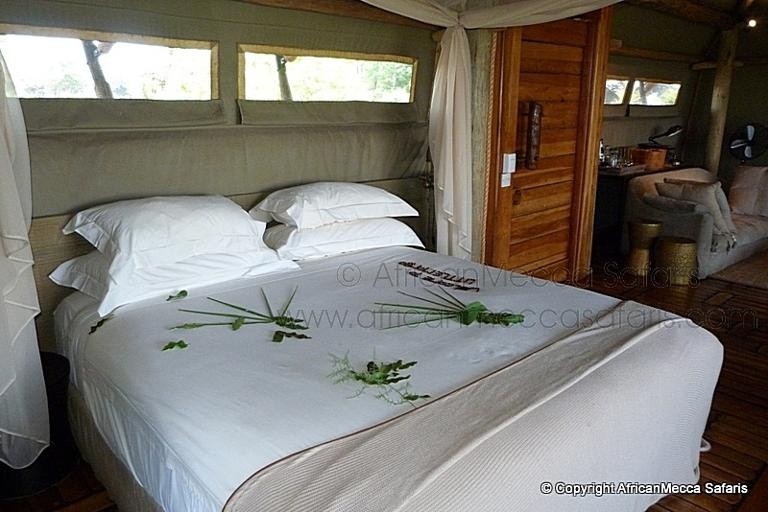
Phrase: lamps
[
  {"left": 647, "top": 125, "right": 686, "bottom": 163},
  {"left": 514, "top": 100, "right": 544, "bottom": 171}
]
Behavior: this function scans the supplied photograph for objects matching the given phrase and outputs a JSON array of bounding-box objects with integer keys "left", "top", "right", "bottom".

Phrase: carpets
[{"left": 709, "top": 252, "right": 767, "bottom": 289}]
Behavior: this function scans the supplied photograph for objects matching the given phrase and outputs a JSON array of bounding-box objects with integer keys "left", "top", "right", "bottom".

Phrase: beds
[{"left": 49, "top": 181, "right": 723, "bottom": 512}]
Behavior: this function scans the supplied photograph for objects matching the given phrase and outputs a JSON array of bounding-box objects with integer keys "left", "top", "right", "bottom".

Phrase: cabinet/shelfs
[{"left": 593, "top": 144, "right": 679, "bottom": 259}]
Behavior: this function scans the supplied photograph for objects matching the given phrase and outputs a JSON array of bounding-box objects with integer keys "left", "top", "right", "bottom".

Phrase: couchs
[{"left": 625, "top": 160, "right": 767, "bottom": 282}]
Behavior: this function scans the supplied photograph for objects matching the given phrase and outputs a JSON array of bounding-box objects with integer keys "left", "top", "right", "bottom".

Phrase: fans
[{"left": 729, "top": 121, "right": 768, "bottom": 165}]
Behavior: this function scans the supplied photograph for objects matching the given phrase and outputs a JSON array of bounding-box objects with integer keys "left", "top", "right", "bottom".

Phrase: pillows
[
  {"left": 729, "top": 167, "right": 767, "bottom": 218},
  {"left": 666, "top": 177, "right": 736, "bottom": 227},
  {"left": 250, "top": 182, "right": 422, "bottom": 229},
  {"left": 50, "top": 251, "right": 300, "bottom": 315},
  {"left": 652, "top": 179, "right": 726, "bottom": 234},
  {"left": 60, "top": 195, "right": 270, "bottom": 284},
  {"left": 263, "top": 216, "right": 426, "bottom": 262}
]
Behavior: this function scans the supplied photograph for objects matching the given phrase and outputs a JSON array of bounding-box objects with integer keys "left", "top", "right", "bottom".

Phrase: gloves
[{"left": 634, "top": 148, "right": 666, "bottom": 168}]
